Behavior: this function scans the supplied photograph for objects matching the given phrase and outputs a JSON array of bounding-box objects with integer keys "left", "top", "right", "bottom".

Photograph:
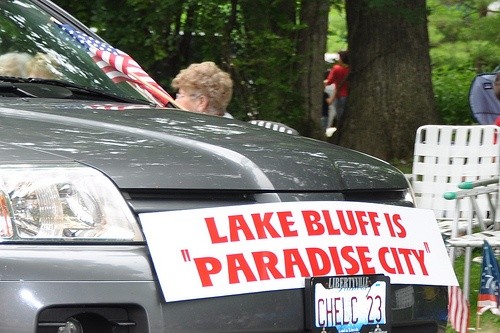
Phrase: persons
[
  {"left": 322, "top": 79, "right": 338, "bottom": 128},
  {"left": 0, "top": 50, "right": 63, "bottom": 80},
  {"left": 171, "top": 61, "right": 233, "bottom": 121},
  {"left": 323, "top": 51, "right": 349, "bottom": 130}
]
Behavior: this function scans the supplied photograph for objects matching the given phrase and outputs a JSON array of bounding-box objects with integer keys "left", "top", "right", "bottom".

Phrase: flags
[
  {"left": 477, "top": 239, "right": 500, "bottom": 315},
  {"left": 64, "top": 33, "right": 130, "bottom": 84},
  {"left": 55, "top": 21, "right": 174, "bottom": 107},
  {"left": 448, "top": 285, "right": 470, "bottom": 333}
]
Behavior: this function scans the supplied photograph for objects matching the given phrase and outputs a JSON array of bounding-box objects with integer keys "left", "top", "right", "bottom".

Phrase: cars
[{"left": 1, "top": 0, "right": 459, "bottom": 333}]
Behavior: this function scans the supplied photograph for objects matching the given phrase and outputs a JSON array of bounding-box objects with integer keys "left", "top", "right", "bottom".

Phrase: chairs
[
  {"left": 442, "top": 182, "right": 499, "bottom": 318},
  {"left": 374, "top": 123, "right": 499, "bottom": 324}
]
